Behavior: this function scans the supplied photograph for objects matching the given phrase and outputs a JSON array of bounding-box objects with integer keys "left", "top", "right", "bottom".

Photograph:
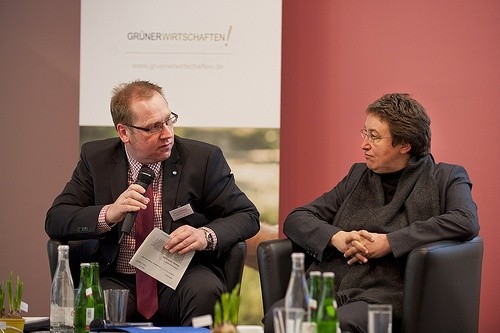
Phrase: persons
[
  {"left": 261, "top": 91, "right": 480, "bottom": 333},
  {"left": 44, "top": 80, "right": 261, "bottom": 329}
]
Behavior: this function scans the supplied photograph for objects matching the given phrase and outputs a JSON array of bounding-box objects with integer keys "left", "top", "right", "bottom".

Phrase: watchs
[{"left": 203, "top": 230, "right": 213, "bottom": 249}]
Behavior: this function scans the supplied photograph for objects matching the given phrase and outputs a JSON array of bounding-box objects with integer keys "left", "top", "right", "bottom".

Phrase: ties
[{"left": 134, "top": 182, "right": 159, "bottom": 319}]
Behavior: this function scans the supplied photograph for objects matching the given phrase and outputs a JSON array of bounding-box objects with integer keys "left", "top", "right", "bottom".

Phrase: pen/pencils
[{"left": 106, "top": 322, "right": 152, "bottom": 327}]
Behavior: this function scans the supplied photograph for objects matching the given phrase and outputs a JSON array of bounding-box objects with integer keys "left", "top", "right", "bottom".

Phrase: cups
[
  {"left": 103, "top": 289, "right": 129, "bottom": 323},
  {"left": 273, "top": 308, "right": 304, "bottom": 333},
  {"left": 367, "top": 304, "right": 392, "bottom": 333}
]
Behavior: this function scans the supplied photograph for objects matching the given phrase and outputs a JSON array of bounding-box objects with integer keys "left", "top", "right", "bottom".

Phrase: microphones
[
  {"left": 121, "top": 167, "right": 155, "bottom": 234},
  {"left": 89, "top": 319, "right": 153, "bottom": 331}
]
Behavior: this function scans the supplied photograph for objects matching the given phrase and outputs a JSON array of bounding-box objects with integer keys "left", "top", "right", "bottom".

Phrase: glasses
[
  {"left": 360, "top": 129, "right": 392, "bottom": 143},
  {"left": 123, "top": 112, "right": 178, "bottom": 134}
]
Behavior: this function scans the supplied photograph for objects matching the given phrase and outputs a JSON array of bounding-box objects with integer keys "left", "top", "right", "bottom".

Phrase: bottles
[
  {"left": 49, "top": 245, "right": 74, "bottom": 333},
  {"left": 317, "top": 272, "right": 337, "bottom": 333},
  {"left": 307, "top": 271, "right": 323, "bottom": 333},
  {"left": 73, "top": 263, "right": 96, "bottom": 333},
  {"left": 285, "top": 253, "right": 310, "bottom": 333},
  {"left": 91, "top": 263, "right": 105, "bottom": 321}
]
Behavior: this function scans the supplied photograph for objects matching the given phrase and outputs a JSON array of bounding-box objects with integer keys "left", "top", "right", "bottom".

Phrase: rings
[{"left": 124, "top": 199, "right": 126, "bottom": 204}]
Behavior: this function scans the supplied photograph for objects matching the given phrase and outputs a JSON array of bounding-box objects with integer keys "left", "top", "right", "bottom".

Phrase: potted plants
[{"left": 0, "top": 271, "right": 24, "bottom": 333}]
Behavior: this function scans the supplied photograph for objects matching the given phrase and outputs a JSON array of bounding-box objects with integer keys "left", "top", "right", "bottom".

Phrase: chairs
[
  {"left": 47, "top": 238, "right": 247, "bottom": 296},
  {"left": 257, "top": 235, "right": 483, "bottom": 333}
]
